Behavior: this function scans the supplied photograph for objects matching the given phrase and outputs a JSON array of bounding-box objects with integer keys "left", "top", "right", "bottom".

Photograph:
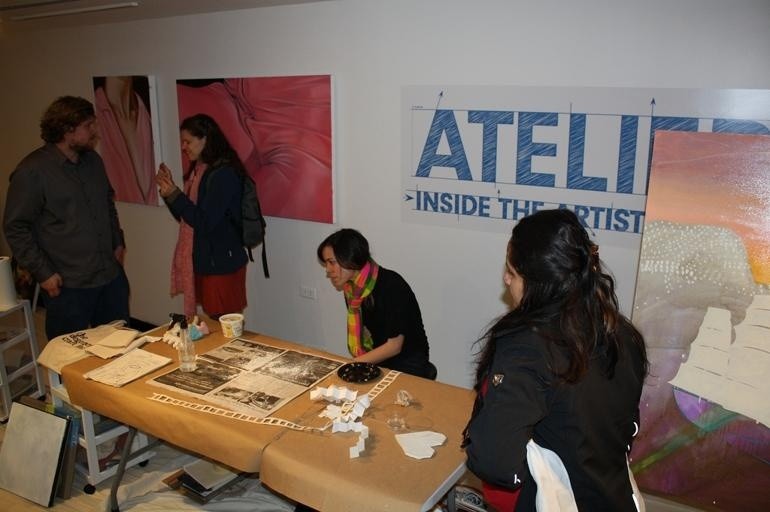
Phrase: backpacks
[{"left": 207, "top": 160, "right": 265, "bottom": 248}]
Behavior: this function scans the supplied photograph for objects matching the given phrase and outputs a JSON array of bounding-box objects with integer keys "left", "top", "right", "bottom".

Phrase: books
[
  {"left": 1, "top": 396, "right": 81, "bottom": 508},
  {"left": 161, "top": 457, "right": 253, "bottom": 505}
]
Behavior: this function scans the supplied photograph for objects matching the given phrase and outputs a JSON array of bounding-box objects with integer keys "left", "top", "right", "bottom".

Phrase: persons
[
  {"left": 317, "top": 229, "right": 429, "bottom": 378},
  {"left": 153, "top": 113, "right": 250, "bottom": 321},
  {"left": 460, "top": 208, "right": 649, "bottom": 512},
  {"left": 94, "top": 76, "right": 159, "bottom": 206},
  {"left": 3, "top": 96, "right": 130, "bottom": 342}
]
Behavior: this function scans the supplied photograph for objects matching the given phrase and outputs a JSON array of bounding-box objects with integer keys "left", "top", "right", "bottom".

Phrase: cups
[{"left": 218, "top": 313, "right": 245, "bottom": 338}]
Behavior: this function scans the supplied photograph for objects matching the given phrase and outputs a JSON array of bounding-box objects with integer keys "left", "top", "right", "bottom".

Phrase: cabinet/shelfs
[{"left": 0, "top": 298, "right": 45, "bottom": 424}]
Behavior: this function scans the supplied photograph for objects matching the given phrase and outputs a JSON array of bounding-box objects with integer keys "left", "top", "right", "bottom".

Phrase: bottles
[{"left": 177, "top": 319, "right": 196, "bottom": 373}]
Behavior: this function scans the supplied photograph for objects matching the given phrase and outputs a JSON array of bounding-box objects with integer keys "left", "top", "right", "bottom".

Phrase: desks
[{"left": 61, "top": 315, "right": 479, "bottom": 512}]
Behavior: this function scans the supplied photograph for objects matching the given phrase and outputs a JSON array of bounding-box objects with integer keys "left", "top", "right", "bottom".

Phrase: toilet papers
[{"left": 0, "top": 257, "right": 18, "bottom": 305}]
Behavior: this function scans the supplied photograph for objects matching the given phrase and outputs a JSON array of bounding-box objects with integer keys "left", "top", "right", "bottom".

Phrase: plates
[{"left": 337, "top": 362, "right": 381, "bottom": 383}]
